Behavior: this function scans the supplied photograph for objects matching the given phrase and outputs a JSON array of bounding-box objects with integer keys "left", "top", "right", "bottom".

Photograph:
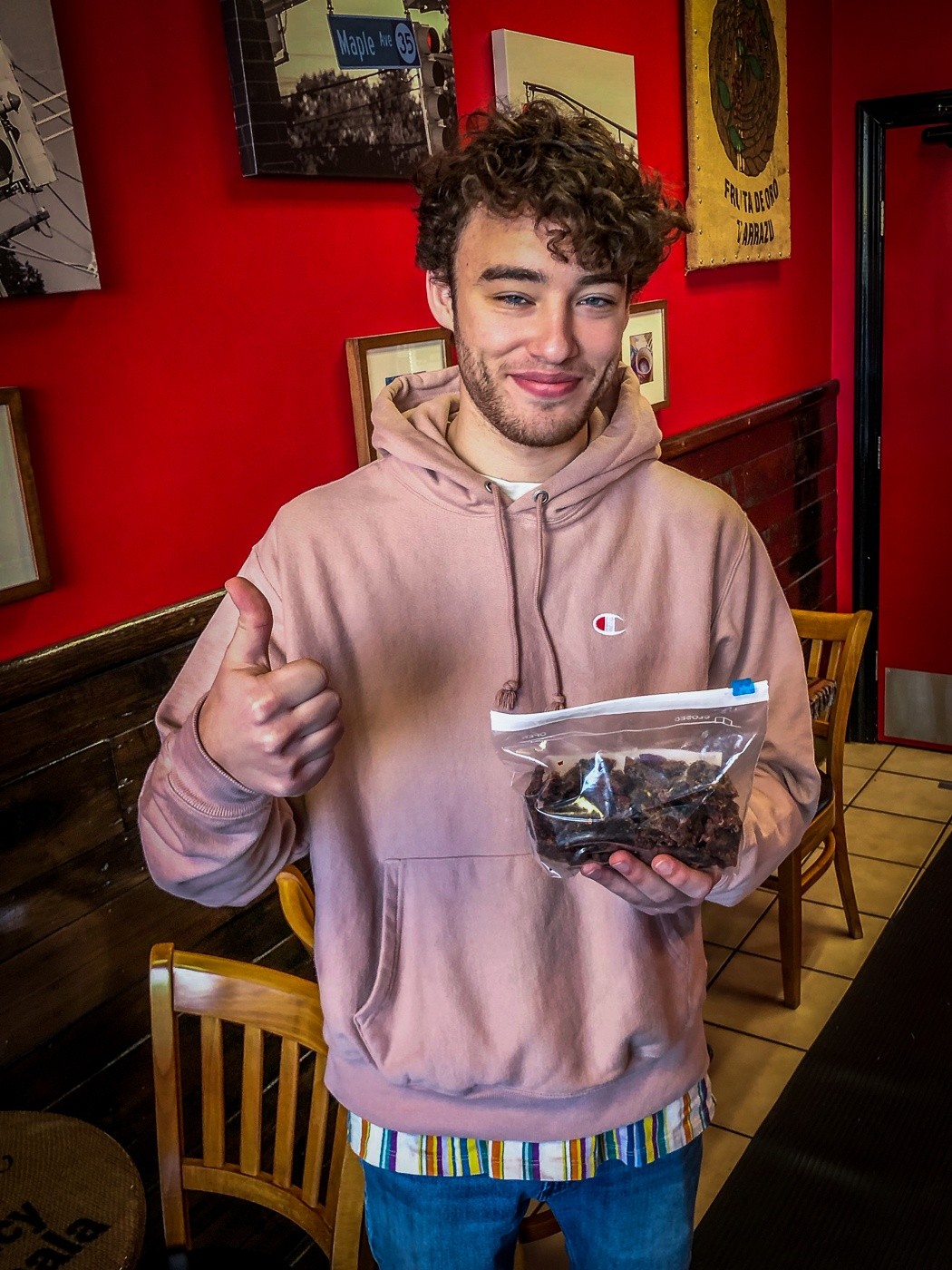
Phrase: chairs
[
  {"left": 760, "top": 605, "right": 873, "bottom": 1011},
  {"left": 133, "top": 936, "right": 366, "bottom": 1270}
]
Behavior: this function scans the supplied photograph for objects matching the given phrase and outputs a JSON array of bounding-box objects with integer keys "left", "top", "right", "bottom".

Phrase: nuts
[{"left": 525, "top": 754, "right": 744, "bottom": 872}]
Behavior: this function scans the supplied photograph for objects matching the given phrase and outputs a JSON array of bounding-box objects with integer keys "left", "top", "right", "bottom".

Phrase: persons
[{"left": 139, "top": 88, "right": 822, "bottom": 1270}]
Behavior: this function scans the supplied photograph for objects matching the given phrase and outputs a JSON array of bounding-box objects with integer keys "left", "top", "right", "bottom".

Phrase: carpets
[{"left": 690, "top": 839, "right": 952, "bottom": 1270}]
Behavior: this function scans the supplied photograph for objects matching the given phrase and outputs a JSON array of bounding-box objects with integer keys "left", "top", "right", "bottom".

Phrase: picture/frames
[
  {"left": 611, "top": 293, "right": 670, "bottom": 415},
  {"left": 0, "top": 387, "right": 55, "bottom": 610},
  {"left": 342, "top": 324, "right": 456, "bottom": 466}
]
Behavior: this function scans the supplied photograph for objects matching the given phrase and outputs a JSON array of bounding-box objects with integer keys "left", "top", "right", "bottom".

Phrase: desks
[{"left": 0, "top": 1106, "right": 153, "bottom": 1270}]
